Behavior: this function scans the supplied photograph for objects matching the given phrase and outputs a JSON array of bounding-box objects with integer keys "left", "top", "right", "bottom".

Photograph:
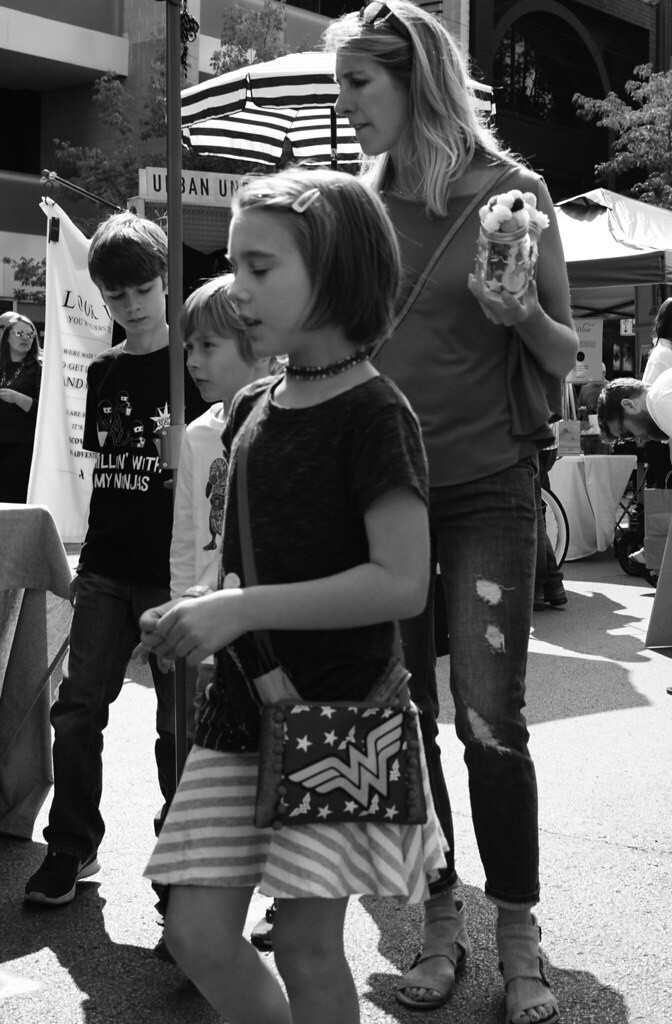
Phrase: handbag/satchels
[{"left": 253, "top": 699, "right": 428, "bottom": 827}]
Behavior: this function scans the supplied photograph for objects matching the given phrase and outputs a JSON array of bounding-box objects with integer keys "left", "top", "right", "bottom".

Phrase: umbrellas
[{"left": 165, "top": 51, "right": 495, "bottom": 172}]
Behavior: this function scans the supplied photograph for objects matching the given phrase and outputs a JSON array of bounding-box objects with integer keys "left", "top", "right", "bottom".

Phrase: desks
[
  {"left": 0, "top": 502, "right": 73, "bottom": 841},
  {"left": 544, "top": 454, "right": 638, "bottom": 561}
]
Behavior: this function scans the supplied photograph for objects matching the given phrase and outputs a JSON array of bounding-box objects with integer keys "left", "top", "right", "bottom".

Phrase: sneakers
[
  {"left": 155, "top": 909, "right": 176, "bottom": 967},
  {"left": 24, "top": 849, "right": 101, "bottom": 904}
]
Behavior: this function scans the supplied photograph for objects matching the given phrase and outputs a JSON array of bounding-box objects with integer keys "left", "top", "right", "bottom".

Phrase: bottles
[{"left": 475, "top": 220, "right": 543, "bottom": 300}]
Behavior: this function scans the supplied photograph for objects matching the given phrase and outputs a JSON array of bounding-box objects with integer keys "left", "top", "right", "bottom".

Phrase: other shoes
[
  {"left": 666, "top": 686, "right": 672, "bottom": 695},
  {"left": 249, "top": 901, "right": 281, "bottom": 950},
  {"left": 533, "top": 580, "right": 566, "bottom": 612}
]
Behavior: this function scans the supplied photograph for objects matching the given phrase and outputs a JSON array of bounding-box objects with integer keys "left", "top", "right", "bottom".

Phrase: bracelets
[{"left": 182, "top": 585, "right": 213, "bottom": 599}]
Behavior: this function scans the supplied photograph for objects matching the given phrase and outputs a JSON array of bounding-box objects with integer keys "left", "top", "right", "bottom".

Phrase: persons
[
  {"left": 0, "top": 313, "right": 41, "bottom": 504},
  {"left": 145, "top": 170, "right": 450, "bottom": 1024},
  {"left": 26, "top": 213, "right": 201, "bottom": 906},
  {"left": 150, "top": 274, "right": 276, "bottom": 969},
  {"left": 313, "top": 0, "right": 582, "bottom": 1024}
]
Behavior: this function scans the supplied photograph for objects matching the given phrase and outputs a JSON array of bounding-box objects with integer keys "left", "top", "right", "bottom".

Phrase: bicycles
[{"left": 541, "top": 485, "right": 570, "bottom": 570}]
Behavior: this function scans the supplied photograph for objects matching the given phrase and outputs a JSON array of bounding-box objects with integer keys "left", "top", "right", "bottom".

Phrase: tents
[{"left": 549, "top": 186, "right": 672, "bottom": 378}]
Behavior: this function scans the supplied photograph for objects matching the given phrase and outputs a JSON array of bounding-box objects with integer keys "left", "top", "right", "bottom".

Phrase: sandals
[
  {"left": 495, "top": 913, "right": 560, "bottom": 1024},
  {"left": 630, "top": 548, "right": 659, "bottom": 588},
  {"left": 394, "top": 899, "right": 471, "bottom": 1009}
]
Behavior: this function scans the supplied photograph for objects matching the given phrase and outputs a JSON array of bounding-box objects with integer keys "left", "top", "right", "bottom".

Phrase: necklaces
[
  {"left": 390, "top": 178, "right": 410, "bottom": 197},
  {"left": 1, "top": 363, "right": 24, "bottom": 388},
  {"left": 284, "top": 347, "right": 366, "bottom": 380}
]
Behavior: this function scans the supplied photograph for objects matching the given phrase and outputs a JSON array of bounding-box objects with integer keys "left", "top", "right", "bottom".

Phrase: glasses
[
  {"left": 617, "top": 415, "right": 636, "bottom": 443},
  {"left": 9, "top": 330, "right": 36, "bottom": 339},
  {"left": 0, "top": 324, "right": 8, "bottom": 330},
  {"left": 358, "top": 0, "right": 415, "bottom": 52}
]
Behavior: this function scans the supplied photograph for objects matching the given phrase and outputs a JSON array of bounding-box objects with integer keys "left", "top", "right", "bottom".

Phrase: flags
[{"left": 24, "top": 201, "right": 112, "bottom": 544}]
